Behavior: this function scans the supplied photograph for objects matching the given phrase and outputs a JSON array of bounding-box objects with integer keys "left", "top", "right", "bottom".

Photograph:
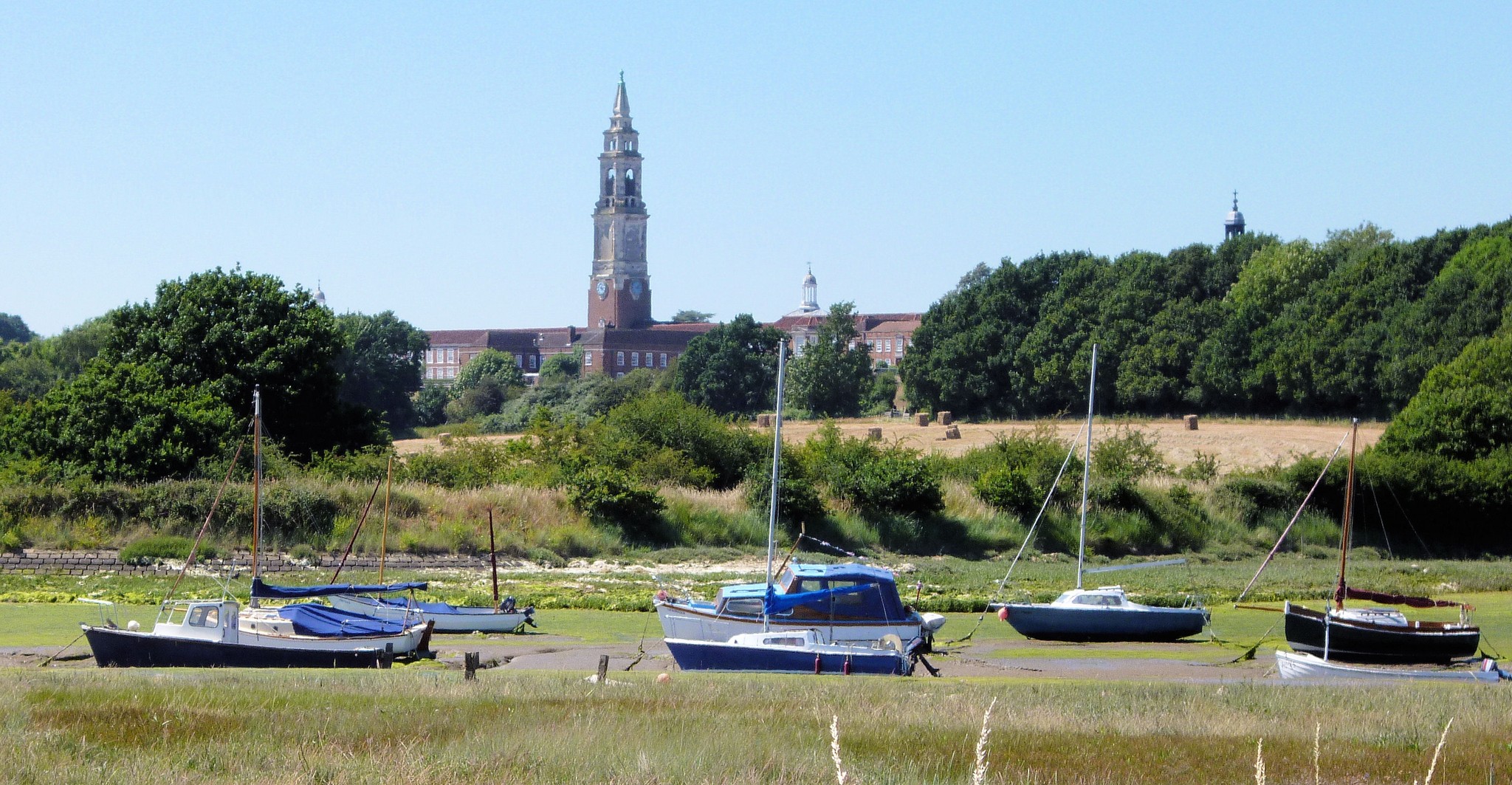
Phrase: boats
[
  {"left": 1275, "top": 597, "right": 1500, "bottom": 684},
  {"left": 76, "top": 600, "right": 384, "bottom": 669},
  {"left": 652, "top": 533, "right": 945, "bottom": 651},
  {"left": 327, "top": 456, "right": 535, "bottom": 633}
]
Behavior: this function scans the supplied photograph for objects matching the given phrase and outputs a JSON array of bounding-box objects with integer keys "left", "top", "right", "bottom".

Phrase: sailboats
[
  {"left": 990, "top": 343, "right": 1212, "bottom": 642},
  {"left": 663, "top": 338, "right": 923, "bottom": 677},
  {"left": 161, "top": 384, "right": 428, "bottom": 656},
  {"left": 1234, "top": 418, "right": 1481, "bottom": 664}
]
